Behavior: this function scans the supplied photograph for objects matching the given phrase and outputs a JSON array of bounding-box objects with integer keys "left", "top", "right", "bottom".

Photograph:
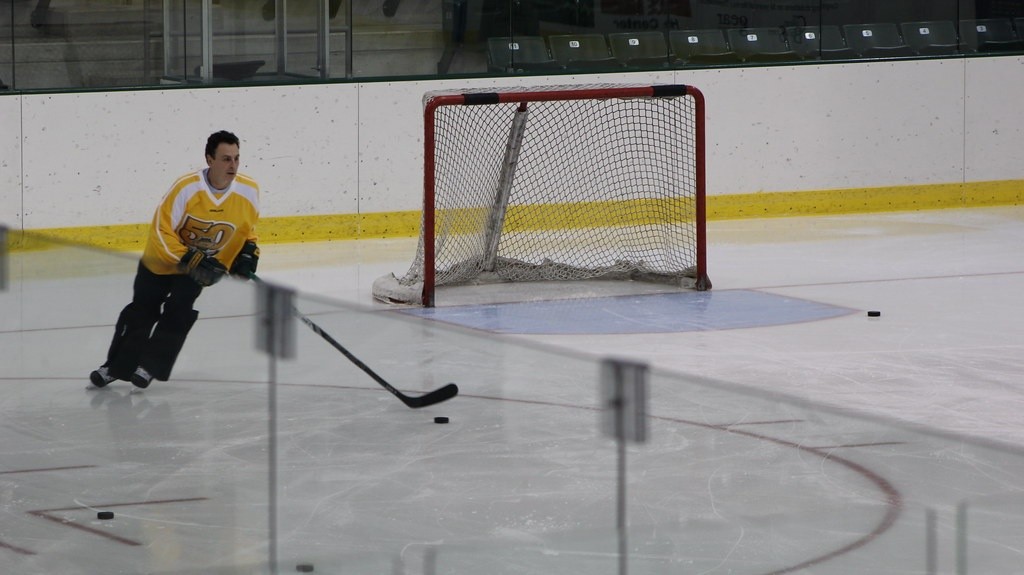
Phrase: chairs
[
  {"left": 785, "top": 26, "right": 854, "bottom": 60},
  {"left": 548, "top": 34, "right": 618, "bottom": 73},
  {"left": 959, "top": 18, "right": 1024, "bottom": 54},
  {"left": 608, "top": 31, "right": 677, "bottom": 70},
  {"left": 900, "top": 20, "right": 967, "bottom": 57},
  {"left": 669, "top": 30, "right": 737, "bottom": 65},
  {"left": 843, "top": 23, "right": 912, "bottom": 58},
  {"left": 486, "top": 36, "right": 558, "bottom": 74},
  {"left": 727, "top": 27, "right": 795, "bottom": 63}
]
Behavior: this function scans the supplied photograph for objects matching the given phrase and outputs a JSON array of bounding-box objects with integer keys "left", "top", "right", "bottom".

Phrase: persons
[{"left": 89, "top": 130, "right": 260, "bottom": 387}]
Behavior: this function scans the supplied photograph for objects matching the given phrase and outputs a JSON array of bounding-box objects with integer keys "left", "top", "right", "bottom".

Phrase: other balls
[
  {"left": 97, "top": 512, "right": 114, "bottom": 520},
  {"left": 867, "top": 311, "right": 881, "bottom": 317},
  {"left": 434, "top": 417, "right": 449, "bottom": 424},
  {"left": 296, "top": 565, "right": 314, "bottom": 572}
]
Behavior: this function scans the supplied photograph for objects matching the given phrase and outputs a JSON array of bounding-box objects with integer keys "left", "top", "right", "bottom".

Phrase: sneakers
[
  {"left": 128, "top": 367, "right": 154, "bottom": 393},
  {"left": 86, "top": 365, "right": 119, "bottom": 389}
]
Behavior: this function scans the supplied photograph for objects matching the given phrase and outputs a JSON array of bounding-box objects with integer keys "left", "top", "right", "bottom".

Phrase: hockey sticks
[{"left": 251, "top": 273, "right": 460, "bottom": 411}]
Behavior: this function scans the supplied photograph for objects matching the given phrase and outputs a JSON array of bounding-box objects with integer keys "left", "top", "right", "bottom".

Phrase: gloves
[
  {"left": 229, "top": 242, "right": 260, "bottom": 280},
  {"left": 177, "top": 249, "right": 227, "bottom": 287}
]
